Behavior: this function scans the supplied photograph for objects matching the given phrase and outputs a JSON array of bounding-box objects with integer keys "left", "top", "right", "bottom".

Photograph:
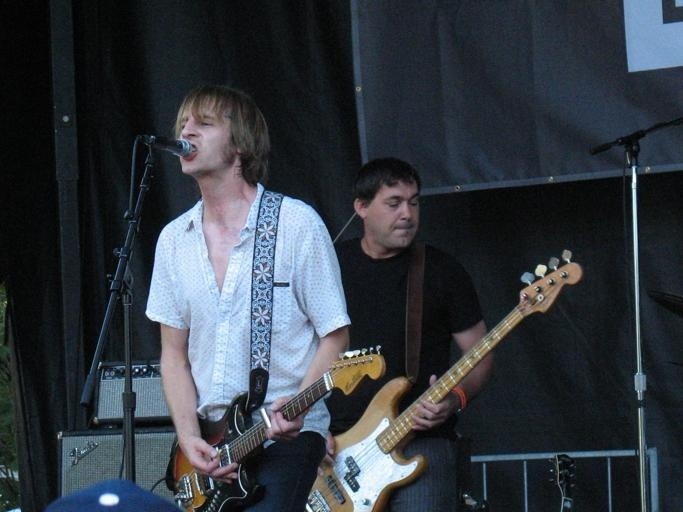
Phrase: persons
[
  {"left": 314, "top": 155, "right": 496, "bottom": 511},
  {"left": 140, "top": 78, "right": 351, "bottom": 512}
]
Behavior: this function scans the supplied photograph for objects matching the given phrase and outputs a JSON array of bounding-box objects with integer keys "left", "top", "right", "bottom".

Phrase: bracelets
[{"left": 452, "top": 387, "right": 468, "bottom": 414}]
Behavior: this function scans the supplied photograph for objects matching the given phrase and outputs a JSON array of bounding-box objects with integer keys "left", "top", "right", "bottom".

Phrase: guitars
[
  {"left": 165, "top": 346, "right": 386, "bottom": 512},
  {"left": 305, "top": 249, "right": 582, "bottom": 511}
]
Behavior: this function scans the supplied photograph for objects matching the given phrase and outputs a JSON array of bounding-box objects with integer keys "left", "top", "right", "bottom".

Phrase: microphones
[{"left": 141, "top": 133, "right": 192, "bottom": 157}]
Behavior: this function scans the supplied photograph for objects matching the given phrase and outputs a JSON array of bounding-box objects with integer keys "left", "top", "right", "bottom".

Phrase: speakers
[{"left": 56, "top": 427, "right": 188, "bottom": 512}]
